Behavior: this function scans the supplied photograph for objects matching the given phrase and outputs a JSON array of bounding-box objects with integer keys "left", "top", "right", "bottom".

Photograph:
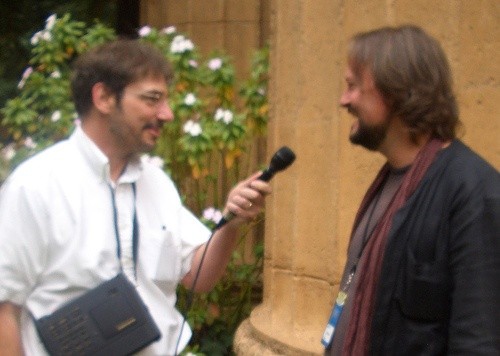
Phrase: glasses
[{"left": 125, "top": 90, "right": 170, "bottom": 106}]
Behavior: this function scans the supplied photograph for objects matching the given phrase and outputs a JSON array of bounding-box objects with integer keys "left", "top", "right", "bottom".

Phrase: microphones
[{"left": 216, "top": 147, "right": 296, "bottom": 228}]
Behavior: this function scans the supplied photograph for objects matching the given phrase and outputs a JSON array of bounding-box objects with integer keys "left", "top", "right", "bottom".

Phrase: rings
[{"left": 244, "top": 201, "right": 253, "bottom": 211}]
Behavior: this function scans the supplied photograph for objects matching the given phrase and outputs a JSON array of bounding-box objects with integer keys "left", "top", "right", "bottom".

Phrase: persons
[
  {"left": 318, "top": 21, "right": 500, "bottom": 356},
  {"left": 0, "top": 35, "right": 271, "bottom": 356}
]
